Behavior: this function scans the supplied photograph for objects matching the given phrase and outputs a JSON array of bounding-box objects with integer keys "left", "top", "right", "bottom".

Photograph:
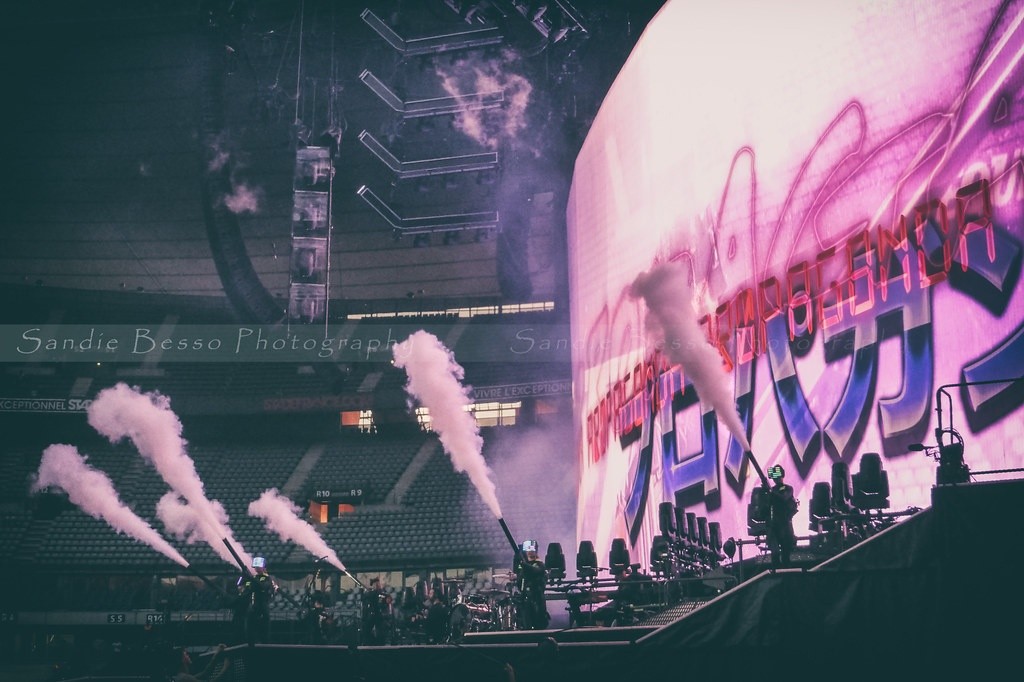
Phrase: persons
[
  {"left": 362, "top": 542, "right": 546, "bottom": 644},
  {"left": 314, "top": 600, "right": 325, "bottom": 643},
  {"left": 173, "top": 644, "right": 230, "bottom": 682},
  {"left": 228, "top": 563, "right": 274, "bottom": 645},
  {"left": 771, "top": 465, "right": 797, "bottom": 569}
]
